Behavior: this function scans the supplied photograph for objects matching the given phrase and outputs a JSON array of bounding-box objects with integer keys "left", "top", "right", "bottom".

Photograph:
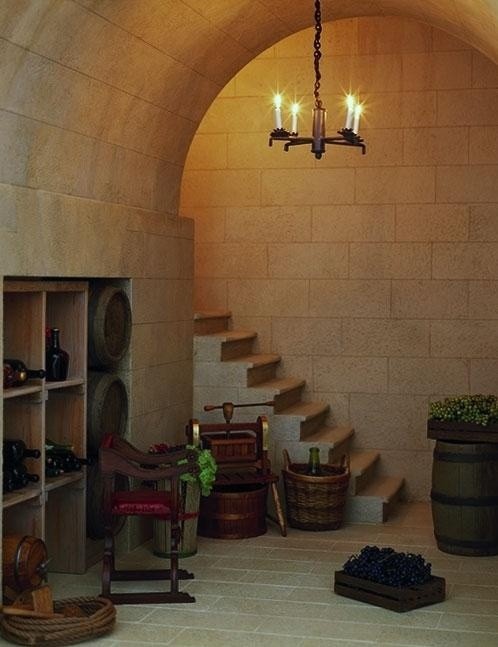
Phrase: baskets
[{"left": 282, "top": 449, "right": 350, "bottom": 531}]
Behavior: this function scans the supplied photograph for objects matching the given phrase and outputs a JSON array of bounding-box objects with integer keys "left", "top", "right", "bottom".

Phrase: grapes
[
  {"left": 342, "top": 545, "right": 432, "bottom": 589},
  {"left": 428, "top": 394, "right": 498, "bottom": 427}
]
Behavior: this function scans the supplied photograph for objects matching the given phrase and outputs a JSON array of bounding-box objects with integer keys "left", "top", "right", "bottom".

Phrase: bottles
[
  {"left": 45, "top": 440, "right": 91, "bottom": 477},
  {"left": 46, "top": 327, "right": 70, "bottom": 381},
  {"left": 3, "top": 438, "right": 41, "bottom": 491},
  {"left": 3, "top": 359, "right": 46, "bottom": 391},
  {"left": 307, "top": 445, "right": 322, "bottom": 477}
]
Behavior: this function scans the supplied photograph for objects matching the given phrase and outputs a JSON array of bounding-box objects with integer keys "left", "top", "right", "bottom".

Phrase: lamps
[{"left": 264, "top": 2, "right": 369, "bottom": 161}]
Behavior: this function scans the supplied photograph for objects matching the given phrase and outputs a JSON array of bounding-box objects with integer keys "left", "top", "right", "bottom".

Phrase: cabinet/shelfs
[{"left": 5, "top": 283, "right": 86, "bottom": 588}]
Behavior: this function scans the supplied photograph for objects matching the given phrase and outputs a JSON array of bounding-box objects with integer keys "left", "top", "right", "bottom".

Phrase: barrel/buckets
[
  {"left": 87, "top": 283, "right": 133, "bottom": 370},
  {"left": 200, "top": 483, "right": 269, "bottom": 539},
  {"left": 429, "top": 441, "right": 497, "bottom": 559},
  {"left": 87, "top": 449, "right": 130, "bottom": 539},
  {"left": 2, "top": 532, "right": 48, "bottom": 602},
  {"left": 87, "top": 371, "right": 129, "bottom": 456}
]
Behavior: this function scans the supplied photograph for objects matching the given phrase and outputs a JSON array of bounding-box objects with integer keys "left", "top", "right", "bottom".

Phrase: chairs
[{"left": 98, "top": 426, "right": 194, "bottom": 607}]
